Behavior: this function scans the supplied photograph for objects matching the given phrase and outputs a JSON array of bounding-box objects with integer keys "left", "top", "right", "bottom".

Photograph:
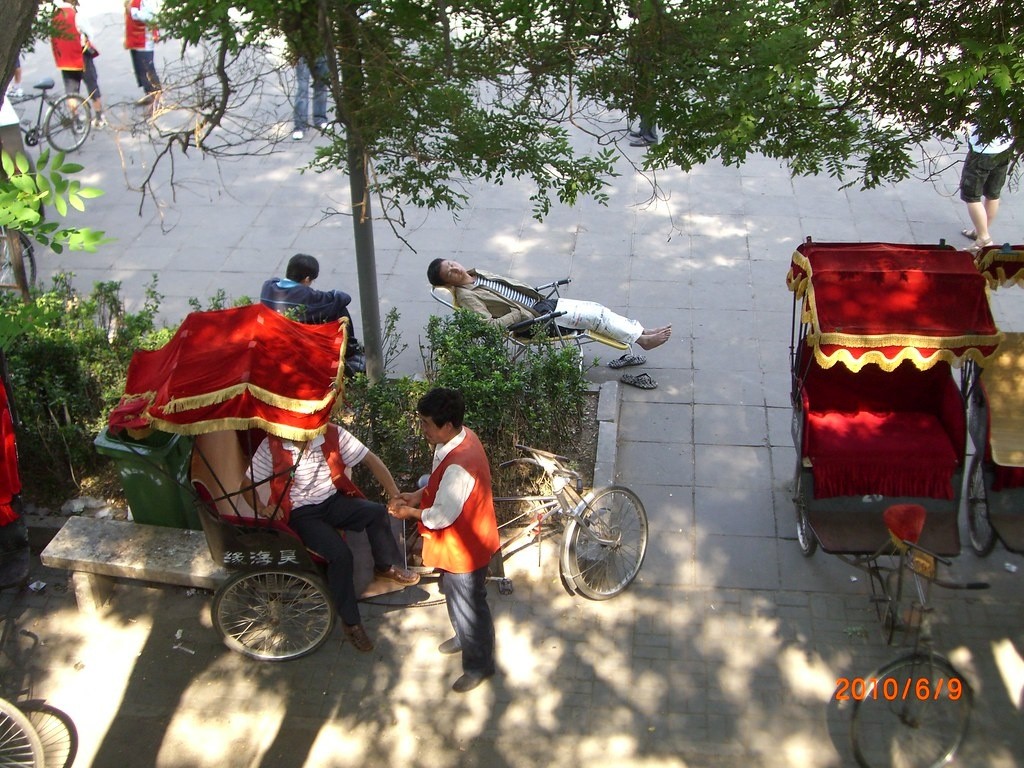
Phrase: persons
[
  {"left": 51, "top": 0, "right": 108, "bottom": 133},
  {"left": 959, "top": 79, "right": 1015, "bottom": 252},
  {"left": 427, "top": 258, "right": 671, "bottom": 350},
  {"left": 125, "top": 0, "right": 161, "bottom": 94},
  {"left": 387, "top": 389, "right": 500, "bottom": 692},
  {"left": 259, "top": 254, "right": 355, "bottom": 338},
  {"left": 292, "top": 56, "right": 332, "bottom": 140},
  {"left": 239, "top": 422, "right": 420, "bottom": 653},
  {"left": 6, "top": 55, "right": 23, "bottom": 97},
  {"left": 629, "top": 114, "right": 658, "bottom": 146}
]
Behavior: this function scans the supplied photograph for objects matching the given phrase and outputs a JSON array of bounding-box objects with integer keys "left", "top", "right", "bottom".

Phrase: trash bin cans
[{"left": 93, "top": 424, "right": 206, "bottom": 533}]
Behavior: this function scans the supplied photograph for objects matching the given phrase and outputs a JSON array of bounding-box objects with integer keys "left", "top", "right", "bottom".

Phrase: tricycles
[
  {"left": 963, "top": 243, "right": 1024, "bottom": 560},
  {"left": 0, "top": 378, "right": 47, "bottom": 768},
  {"left": 0, "top": 96, "right": 45, "bottom": 306},
  {"left": 110, "top": 303, "right": 649, "bottom": 666},
  {"left": 784, "top": 232, "right": 1004, "bottom": 767}
]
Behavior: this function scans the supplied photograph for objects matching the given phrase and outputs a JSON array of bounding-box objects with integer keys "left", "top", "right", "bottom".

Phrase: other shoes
[
  {"left": 12, "top": 83, "right": 22, "bottom": 95},
  {"left": 438, "top": 638, "right": 461, "bottom": 654},
  {"left": 321, "top": 123, "right": 332, "bottom": 129},
  {"left": 292, "top": 131, "right": 303, "bottom": 139},
  {"left": 453, "top": 674, "right": 481, "bottom": 692},
  {"left": 95, "top": 120, "right": 107, "bottom": 126},
  {"left": 76, "top": 126, "right": 84, "bottom": 133}
]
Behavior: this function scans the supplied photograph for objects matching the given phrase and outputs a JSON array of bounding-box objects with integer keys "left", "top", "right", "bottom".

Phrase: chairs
[{"left": 431, "top": 276, "right": 630, "bottom": 379}]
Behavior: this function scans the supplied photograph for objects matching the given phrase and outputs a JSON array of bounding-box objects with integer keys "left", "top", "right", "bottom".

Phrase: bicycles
[{"left": 4, "top": 78, "right": 96, "bottom": 156}]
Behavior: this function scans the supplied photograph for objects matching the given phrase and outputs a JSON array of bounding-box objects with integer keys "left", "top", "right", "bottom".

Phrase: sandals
[
  {"left": 963, "top": 237, "right": 992, "bottom": 253},
  {"left": 961, "top": 229, "right": 977, "bottom": 240}
]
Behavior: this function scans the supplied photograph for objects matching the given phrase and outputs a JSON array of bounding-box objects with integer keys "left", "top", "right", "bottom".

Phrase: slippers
[
  {"left": 609, "top": 352, "right": 646, "bottom": 369},
  {"left": 621, "top": 372, "right": 658, "bottom": 389}
]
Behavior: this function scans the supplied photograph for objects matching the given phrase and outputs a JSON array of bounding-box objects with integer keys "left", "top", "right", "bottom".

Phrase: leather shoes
[
  {"left": 374, "top": 564, "right": 420, "bottom": 586},
  {"left": 630, "top": 137, "right": 658, "bottom": 147},
  {"left": 630, "top": 131, "right": 640, "bottom": 136},
  {"left": 341, "top": 622, "right": 373, "bottom": 652}
]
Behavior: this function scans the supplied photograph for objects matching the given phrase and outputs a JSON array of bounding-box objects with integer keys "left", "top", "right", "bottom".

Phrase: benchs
[
  {"left": 40, "top": 516, "right": 249, "bottom": 620},
  {"left": 191, "top": 426, "right": 350, "bottom": 576},
  {"left": 794, "top": 339, "right": 967, "bottom": 497},
  {"left": 977, "top": 333, "right": 1024, "bottom": 493}
]
